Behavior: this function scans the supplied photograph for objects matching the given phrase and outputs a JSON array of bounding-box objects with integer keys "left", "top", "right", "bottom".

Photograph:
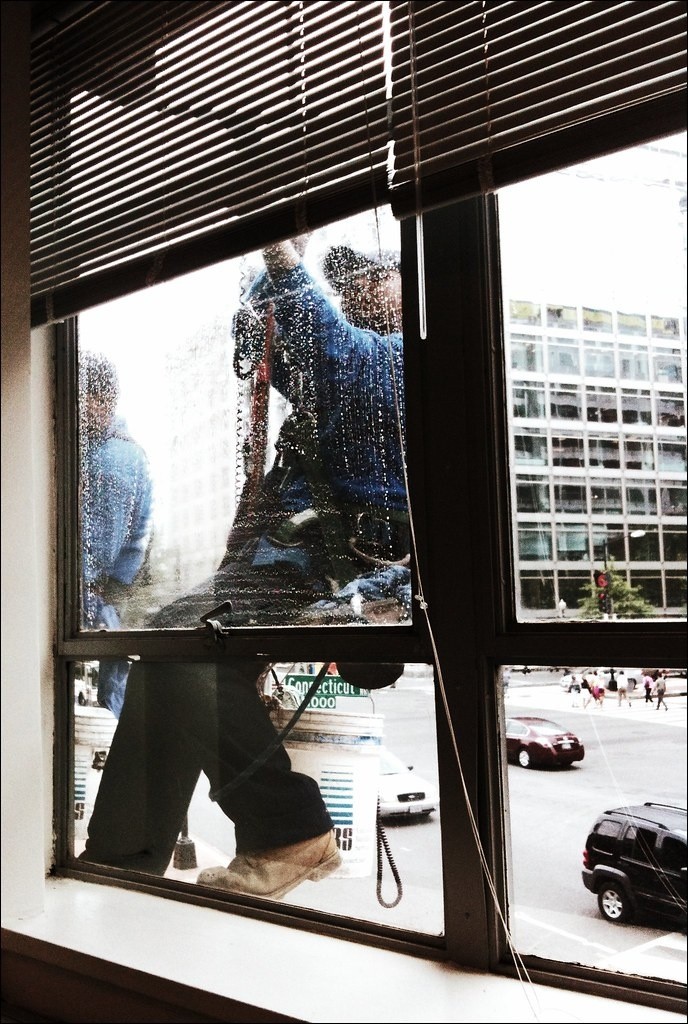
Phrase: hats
[{"left": 321, "top": 243, "right": 399, "bottom": 293}]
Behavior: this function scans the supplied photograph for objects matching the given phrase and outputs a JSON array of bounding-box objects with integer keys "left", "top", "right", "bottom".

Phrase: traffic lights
[{"left": 594, "top": 572, "right": 612, "bottom": 613}]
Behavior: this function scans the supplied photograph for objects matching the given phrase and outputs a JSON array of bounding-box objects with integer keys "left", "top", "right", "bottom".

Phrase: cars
[
  {"left": 380, "top": 749, "right": 434, "bottom": 820},
  {"left": 595, "top": 666, "right": 646, "bottom": 692},
  {"left": 557, "top": 667, "right": 588, "bottom": 689},
  {"left": 503, "top": 716, "right": 585, "bottom": 770}
]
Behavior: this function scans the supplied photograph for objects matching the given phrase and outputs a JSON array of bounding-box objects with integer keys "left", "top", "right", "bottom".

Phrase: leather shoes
[{"left": 195, "top": 829, "right": 341, "bottom": 903}]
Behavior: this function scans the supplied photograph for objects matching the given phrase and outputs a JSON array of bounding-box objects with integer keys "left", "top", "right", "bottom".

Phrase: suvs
[{"left": 582, "top": 802, "right": 688, "bottom": 927}]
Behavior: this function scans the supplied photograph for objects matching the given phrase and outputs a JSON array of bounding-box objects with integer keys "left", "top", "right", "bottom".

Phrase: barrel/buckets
[
  {"left": 267, "top": 709, "right": 384, "bottom": 881},
  {"left": 73, "top": 706, "right": 123, "bottom": 860}
]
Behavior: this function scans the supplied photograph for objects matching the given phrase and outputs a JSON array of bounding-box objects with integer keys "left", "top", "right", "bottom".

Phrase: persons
[
  {"left": 558, "top": 598, "right": 567, "bottom": 617},
  {"left": 585, "top": 671, "right": 607, "bottom": 705},
  {"left": 616, "top": 671, "right": 630, "bottom": 705},
  {"left": 78, "top": 228, "right": 411, "bottom": 900},
  {"left": 79, "top": 351, "right": 153, "bottom": 631},
  {"left": 650, "top": 673, "right": 668, "bottom": 710},
  {"left": 644, "top": 674, "right": 654, "bottom": 702},
  {"left": 503, "top": 667, "right": 511, "bottom": 694}
]
